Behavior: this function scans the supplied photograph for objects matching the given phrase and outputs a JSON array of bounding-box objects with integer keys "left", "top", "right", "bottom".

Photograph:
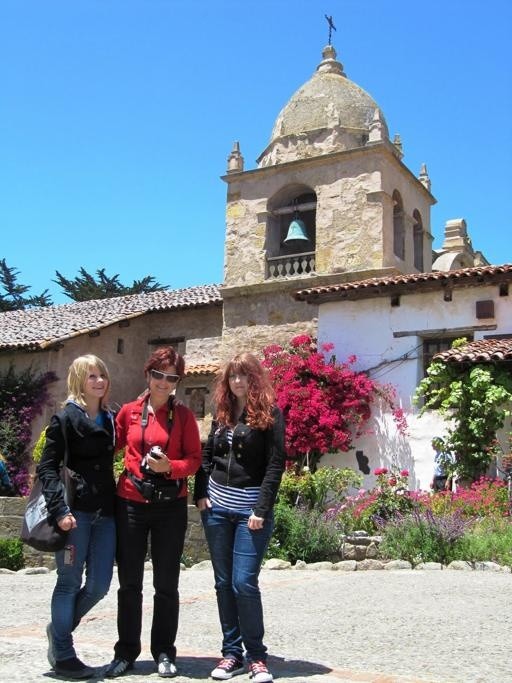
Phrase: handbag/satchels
[
  {"left": 138, "top": 477, "right": 180, "bottom": 504},
  {"left": 18, "top": 462, "right": 86, "bottom": 551}
]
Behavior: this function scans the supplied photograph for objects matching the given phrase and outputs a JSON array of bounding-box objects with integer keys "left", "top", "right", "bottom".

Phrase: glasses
[{"left": 148, "top": 368, "right": 183, "bottom": 385}]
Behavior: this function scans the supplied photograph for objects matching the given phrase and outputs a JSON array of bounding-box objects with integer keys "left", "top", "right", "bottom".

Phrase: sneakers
[
  {"left": 155, "top": 653, "right": 178, "bottom": 677},
  {"left": 46, "top": 622, "right": 96, "bottom": 678},
  {"left": 101, "top": 656, "right": 134, "bottom": 676},
  {"left": 247, "top": 657, "right": 274, "bottom": 683},
  {"left": 210, "top": 655, "right": 245, "bottom": 680}
]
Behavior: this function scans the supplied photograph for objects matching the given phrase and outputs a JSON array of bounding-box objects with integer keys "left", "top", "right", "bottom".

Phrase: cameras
[{"left": 139, "top": 444, "right": 168, "bottom": 473}]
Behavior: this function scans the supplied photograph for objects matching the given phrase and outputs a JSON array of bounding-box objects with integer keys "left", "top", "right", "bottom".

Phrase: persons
[
  {"left": 37, "top": 354, "right": 185, "bottom": 683},
  {"left": 429, "top": 436, "right": 453, "bottom": 491},
  {"left": 102, "top": 347, "right": 202, "bottom": 678},
  {"left": 193, "top": 350, "right": 285, "bottom": 683}
]
